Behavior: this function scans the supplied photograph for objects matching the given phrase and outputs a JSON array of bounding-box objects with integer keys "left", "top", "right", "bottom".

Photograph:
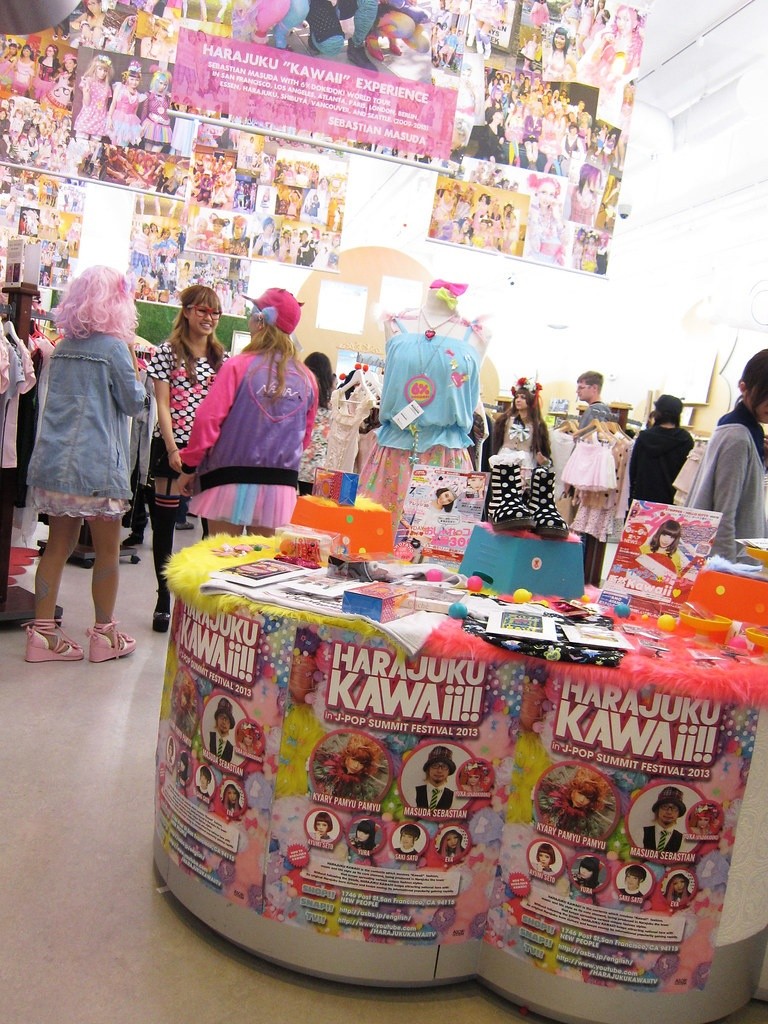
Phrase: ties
[
  {"left": 429, "top": 787, "right": 439, "bottom": 809},
  {"left": 657, "top": 830, "right": 669, "bottom": 853},
  {"left": 217, "top": 739, "right": 223, "bottom": 758}
]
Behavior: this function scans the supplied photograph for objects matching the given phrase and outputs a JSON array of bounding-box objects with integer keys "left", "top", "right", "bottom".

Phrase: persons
[
  {"left": 494, "top": 380, "right": 552, "bottom": 464},
  {"left": 359, "top": 279, "right": 491, "bottom": 514},
  {"left": 299, "top": 349, "right": 338, "bottom": 496},
  {"left": 164, "top": 736, "right": 192, "bottom": 787},
  {"left": 439, "top": 828, "right": 465, "bottom": 863},
  {"left": 577, "top": 856, "right": 600, "bottom": 890},
  {"left": 575, "top": 370, "right": 618, "bottom": 433},
  {"left": 629, "top": 395, "right": 695, "bottom": 504},
  {"left": 686, "top": 348, "right": 768, "bottom": 567},
  {"left": 217, "top": 783, "right": 242, "bottom": 816},
  {"left": 195, "top": 765, "right": 213, "bottom": 798},
  {"left": 203, "top": 695, "right": 236, "bottom": 763},
  {"left": 0, "top": 1, "right": 347, "bottom": 316},
  {"left": 644, "top": 519, "right": 683, "bottom": 575},
  {"left": 668, "top": 872, "right": 692, "bottom": 905},
  {"left": 349, "top": 818, "right": 378, "bottom": 853},
  {"left": 305, "top": 810, "right": 334, "bottom": 843},
  {"left": 635, "top": 786, "right": 688, "bottom": 854},
  {"left": 178, "top": 1, "right": 450, "bottom": 173},
  {"left": 531, "top": 842, "right": 557, "bottom": 876},
  {"left": 122, "top": 285, "right": 319, "bottom": 636},
  {"left": 409, "top": 746, "right": 456, "bottom": 809},
  {"left": 398, "top": 825, "right": 421, "bottom": 856},
  {"left": 400, "top": 484, "right": 465, "bottom": 559},
  {"left": 24, "top": 262, "right": 147, "bottom": 662},
  {"left": 424, "top": 0, "right": 646, "bottom": 279},
  {"left": 619, "top": 865, "right": 647, "bottom": 898}
]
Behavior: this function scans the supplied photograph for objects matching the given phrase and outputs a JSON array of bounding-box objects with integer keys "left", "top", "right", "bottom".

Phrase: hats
[
  {"left": 651, "top": 787, "right": 686, "bottom": 818},
  {"left": 654, "top": 395, "right": 683, "bottom": 417},
  {"left": 214, "top": 697, "right": 236, "bottom": 729},
  {"left": 241, "top": 286, "right": 304, "bottom": 334},
  {"left": 422, "top": 746, "right": 456, "bottom": 777}
]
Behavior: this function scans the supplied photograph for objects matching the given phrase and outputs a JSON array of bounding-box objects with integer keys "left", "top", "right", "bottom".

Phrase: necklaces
[{"left": 422, "top": 309, "right": 459, "bottom": 340}]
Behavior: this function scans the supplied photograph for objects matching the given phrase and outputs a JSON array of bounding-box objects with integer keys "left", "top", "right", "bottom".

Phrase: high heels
[
  {"left": 87, "top": 623, "right": 135, "bottom": 663},
  {"left": 22, "top": 619, "right": 85, "bottom": 663}
]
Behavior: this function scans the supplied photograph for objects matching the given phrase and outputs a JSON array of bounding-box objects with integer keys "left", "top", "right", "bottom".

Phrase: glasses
[
  {"left": 219, "top": 714, "right": 231, "bottom": 722},
  {"left": 186, "top": 304, "right": 223, "bottom": 321},
  {"left": 659, "top": 803, "right": 679, "bottom": 813},
  {"left": 429, "top": 762, "right": 448, "bottom": 772}
]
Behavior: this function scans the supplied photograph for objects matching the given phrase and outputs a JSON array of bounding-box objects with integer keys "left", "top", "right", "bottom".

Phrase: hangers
[
  {"left": 556, "top": 408, "right": 631, "bottom": 450},
  {"left": 0, "top": 304, "right": 57, "bottom": 354},
  {"left": 335, "top": 352, "right": 384, "bottom": 411}
]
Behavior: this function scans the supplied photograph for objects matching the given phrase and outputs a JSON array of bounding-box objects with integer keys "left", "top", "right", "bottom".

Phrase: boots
[
  {"left": 529, "top": 466, "right": 569, "bottom": 540},
  {"left": 490, "top": 462, "right": 534, "bottom": 529}
]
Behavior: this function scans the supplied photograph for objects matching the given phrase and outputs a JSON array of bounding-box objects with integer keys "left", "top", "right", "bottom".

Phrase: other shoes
[
  {"left": 152, "top": 610, "right": 170, "bottom": 633},
  {"left": 121, "top": 533, "right": 143, "bottom": 547},
  {"left": 176, "top": 520, "right": 194, "bottom": 532}
]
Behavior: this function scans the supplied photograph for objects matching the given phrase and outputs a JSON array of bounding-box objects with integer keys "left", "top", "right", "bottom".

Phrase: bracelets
[{"left": 167, "top": 449, "right": 178, "bottom": 457}]
